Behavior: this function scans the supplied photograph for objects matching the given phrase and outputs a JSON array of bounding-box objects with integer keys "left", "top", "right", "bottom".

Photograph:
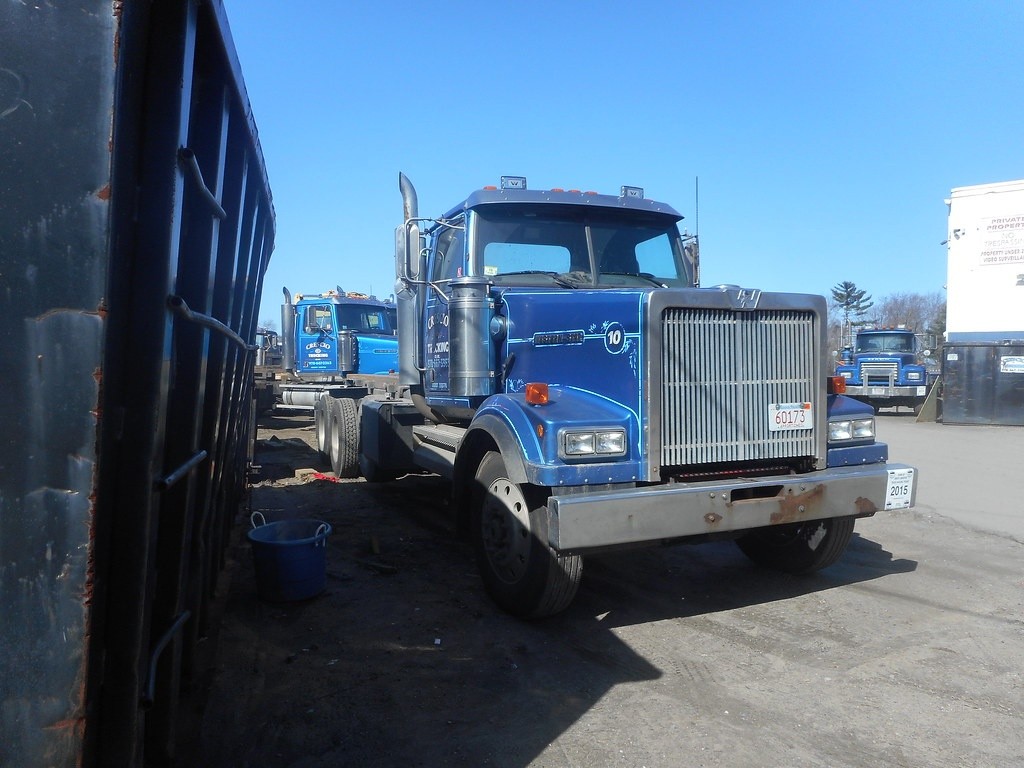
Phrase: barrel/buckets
[{"left": 247, "top": 512, "right": 332, "bottom": 602}]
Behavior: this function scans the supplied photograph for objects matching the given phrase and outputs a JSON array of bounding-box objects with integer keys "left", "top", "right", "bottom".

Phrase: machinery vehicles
[
  {"left": 250, "top": 286, "right": 400, "bottom": 414},
  {"left": 831, "top": 329, "right": 934, "bottom": 417},
  {"left": 313, "top": 168, "right": 918, "bottom": 623}
]
